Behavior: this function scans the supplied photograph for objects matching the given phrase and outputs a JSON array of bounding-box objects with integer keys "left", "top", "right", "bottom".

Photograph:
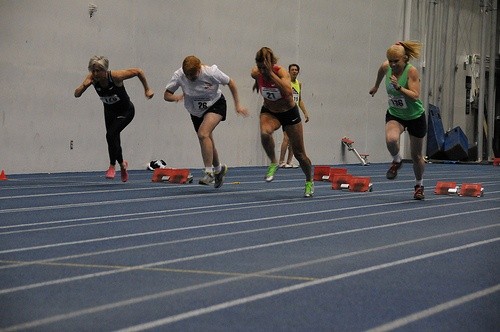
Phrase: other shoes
[
  {"left": 278, "top": 161, "right": 292, "bottom": 168},
  {"left": 288, "top": 162, "right": 299, "bottom": 168}
]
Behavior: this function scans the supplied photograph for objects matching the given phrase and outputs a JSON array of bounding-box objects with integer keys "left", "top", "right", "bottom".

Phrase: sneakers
[
  {"left": 198, "top": 170, "right": 215, "bottom": 185},
  {"left": 386, "top": 158, "right": 403, "bottom": 180},
  {"left": 264, "top": 162, "right": 280, "bottom": 182},
  {"left": 120, "top": 160, "right": 128, "bottom": 183},
  {"left": 304, "top": 179, "right": 314, "bottom": 198},
  {"left": 213, "top": 164, "right": 228, "bottom": 188},
  {"left": 105, "top": 164, "right": 115, "bottom": 179},
  {"left": 413, "top": 183, "right": 426, "bottom": 200}
]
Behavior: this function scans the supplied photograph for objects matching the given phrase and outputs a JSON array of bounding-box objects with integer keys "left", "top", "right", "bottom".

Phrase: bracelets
[
  {"left": 83, "top": 83, "right": 87, "bottom": 89},
  {"left": 396, "top": 86, "right": 401, "bottom": 91}
]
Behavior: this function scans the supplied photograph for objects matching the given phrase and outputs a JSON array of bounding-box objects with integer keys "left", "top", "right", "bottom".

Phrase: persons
[
  {"left": 279, "top": 63, "right": 310, "bottom": 169},
  {"left": 369, "top": 40, "right": 428, "bottom": 200},
  {"left": 164, "top": 55, "right": 250, "bottom": 189},
  {"left": 251, "top": 47, "right": 315, "bottom": 198},
  {"left": 74, "top": 56, "right": 155, "bottom": 182}
]
